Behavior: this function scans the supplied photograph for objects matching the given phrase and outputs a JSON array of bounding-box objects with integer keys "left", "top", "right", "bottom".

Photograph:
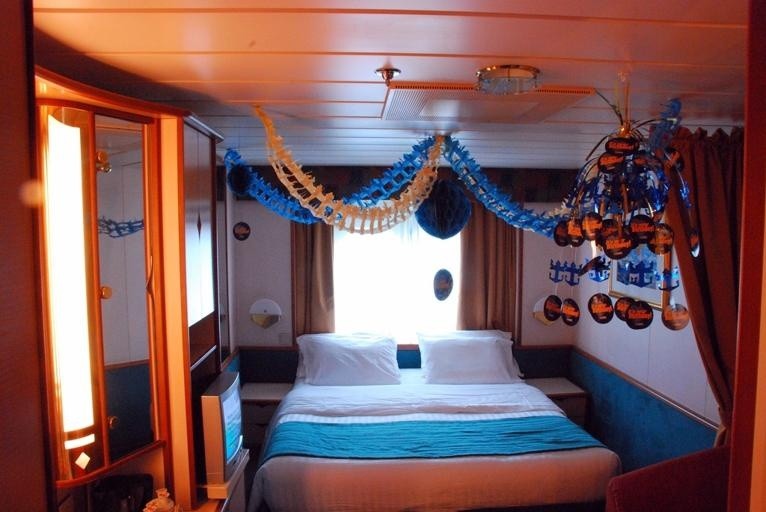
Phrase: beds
[{"left": 264, "top": 328, "right": 620, "bottom": 512}]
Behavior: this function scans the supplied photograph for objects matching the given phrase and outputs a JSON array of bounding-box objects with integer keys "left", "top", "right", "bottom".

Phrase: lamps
[{"left": 472, "top": 64, "right": 541, "bottom": 99}]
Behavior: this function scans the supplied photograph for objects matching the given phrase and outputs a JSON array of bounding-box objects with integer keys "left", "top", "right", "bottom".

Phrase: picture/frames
[{"left": 608, "top": 250, "right": 672, "bottom": 311}]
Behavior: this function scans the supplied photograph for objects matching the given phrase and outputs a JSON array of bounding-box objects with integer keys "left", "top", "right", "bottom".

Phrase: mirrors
[{"left": 91, "top": 111, "right": 156, "bottom": 466}]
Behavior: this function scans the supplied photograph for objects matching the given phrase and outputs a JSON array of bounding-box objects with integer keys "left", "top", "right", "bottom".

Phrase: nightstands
[
  {"left": 524, "top": 377, "right": 588, "bottom": 425},
  {"left": 239, "top": 382, "right": 294, "bottom": 441}
]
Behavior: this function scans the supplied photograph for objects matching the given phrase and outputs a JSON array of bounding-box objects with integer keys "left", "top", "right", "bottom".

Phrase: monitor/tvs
[{"left": 201, "top": 370, "right": 244, "bottom": 483}]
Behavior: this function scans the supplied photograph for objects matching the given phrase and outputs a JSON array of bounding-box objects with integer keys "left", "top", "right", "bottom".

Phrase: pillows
[{"left": 294, "top": 330, "right": 522, "bottom": 385}]
